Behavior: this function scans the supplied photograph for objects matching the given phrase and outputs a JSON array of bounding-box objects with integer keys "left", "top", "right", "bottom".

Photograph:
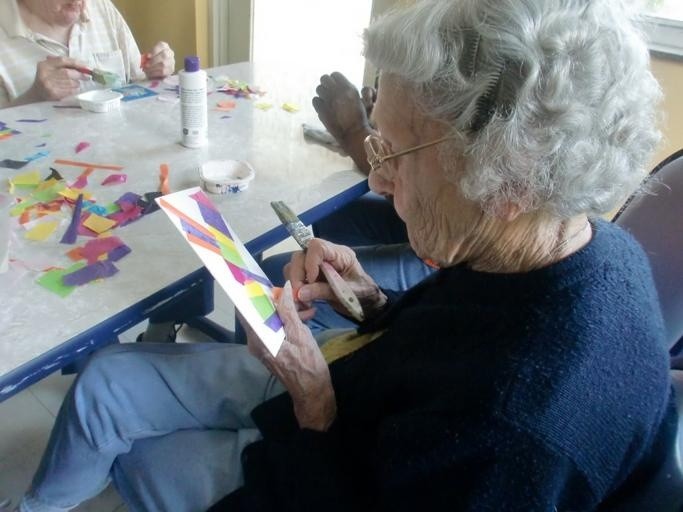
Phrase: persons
[
  {"left": 10, "top": 0, "right": 672, "bottom": 512},
  {"left": 257, "top": 70, "right": 443, "bottom": 339},
  {"left": 0, "top": 0, "right": 214, "bottom": 375}
]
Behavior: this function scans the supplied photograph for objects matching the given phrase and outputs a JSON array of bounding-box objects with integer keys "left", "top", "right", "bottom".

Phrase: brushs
[
  {"left": 65, "top": 64, "right": 116, "bottom": 86},
  {"left": 270, "top": 200, "right": 364, "bottom": 322}
]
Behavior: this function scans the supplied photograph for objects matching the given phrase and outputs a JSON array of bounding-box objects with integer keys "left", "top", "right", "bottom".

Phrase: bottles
[{"left": 178, "top": 55, "right": 209, "bottom": 149}]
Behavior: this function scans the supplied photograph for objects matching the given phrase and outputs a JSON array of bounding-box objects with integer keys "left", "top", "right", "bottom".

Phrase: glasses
[{"left": 364, "top": 135, "right": 458, "bottom": 172}]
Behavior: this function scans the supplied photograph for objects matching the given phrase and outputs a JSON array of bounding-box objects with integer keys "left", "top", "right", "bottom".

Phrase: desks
[{"left": 0, "top": 61, "right": 368, "bottom": 404}]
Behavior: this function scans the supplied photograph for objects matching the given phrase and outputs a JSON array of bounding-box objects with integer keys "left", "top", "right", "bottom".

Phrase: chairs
[
  {"left": 610, "top": 153, "right": 683, "bottom": 370},
  {"left": 605, "top": 369, "right": 683, "bottom": 512}
]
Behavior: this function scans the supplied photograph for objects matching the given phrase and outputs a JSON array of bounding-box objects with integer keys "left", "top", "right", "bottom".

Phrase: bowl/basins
[
  {"left": 200, "top": 158, "right": 255, "bottom": 196},
  {"left": 75, "top": 89, "right": 123, "bottom": 113}
]
